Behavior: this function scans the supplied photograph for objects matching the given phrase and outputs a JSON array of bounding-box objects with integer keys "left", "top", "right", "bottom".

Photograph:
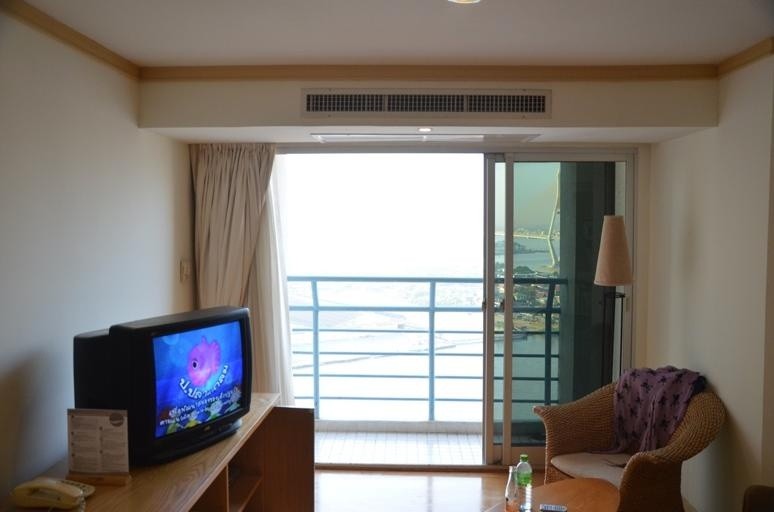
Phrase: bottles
[
  {"left": 515, "top": 451, "right": 535, "bottom": 512},
  {"left": 505, "top": 466, "right": 519, "bottom": 512}
]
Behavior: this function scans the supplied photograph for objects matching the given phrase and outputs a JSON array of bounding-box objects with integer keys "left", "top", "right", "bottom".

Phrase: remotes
[{"left": 539, "top": 504, "right": 568, "bottom": 512}]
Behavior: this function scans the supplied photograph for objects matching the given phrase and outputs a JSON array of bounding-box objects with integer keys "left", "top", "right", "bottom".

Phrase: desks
[
  {"left": 484, "top": 475, "right": 620, "bottom": 512},
  {"left": 0, "top": 392, "right": 315, "bottom": 512}
]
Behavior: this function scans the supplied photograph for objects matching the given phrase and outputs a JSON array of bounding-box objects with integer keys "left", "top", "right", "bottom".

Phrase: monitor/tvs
[{"left": 71, "top": 306, "right": 253, "bottom": 465}]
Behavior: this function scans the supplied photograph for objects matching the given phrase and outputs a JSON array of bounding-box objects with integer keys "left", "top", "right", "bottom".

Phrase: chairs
[{"left": 533, "top": 369, "right": 725, "bottom": 512}]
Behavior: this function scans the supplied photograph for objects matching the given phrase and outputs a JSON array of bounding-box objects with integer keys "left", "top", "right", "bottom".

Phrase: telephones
[{"left": 10, "top": 477, "right": 94, "bottom": 512}]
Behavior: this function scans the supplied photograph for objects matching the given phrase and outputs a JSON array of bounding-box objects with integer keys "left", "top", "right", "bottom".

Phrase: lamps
[{"left": 594, "top": 214, "right": 635, "bottom": 390}]
